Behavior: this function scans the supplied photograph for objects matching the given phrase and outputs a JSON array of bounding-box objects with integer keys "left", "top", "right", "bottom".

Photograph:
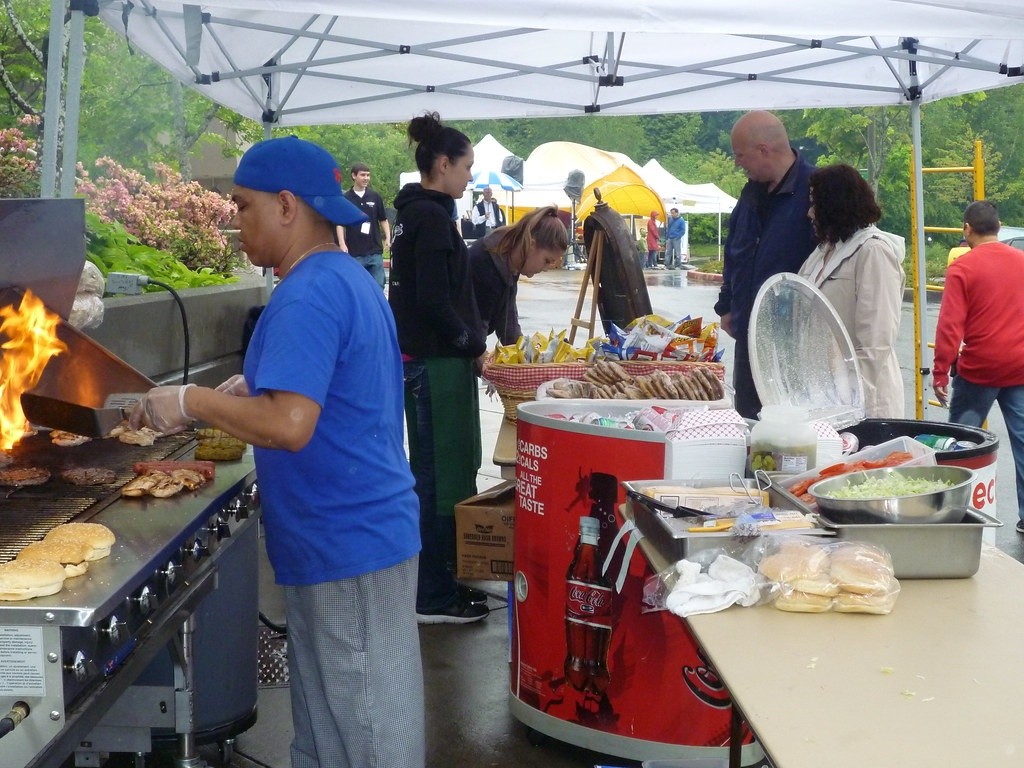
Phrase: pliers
[{"left": 729, "top": 468, "right": 772, "bottom": 507}]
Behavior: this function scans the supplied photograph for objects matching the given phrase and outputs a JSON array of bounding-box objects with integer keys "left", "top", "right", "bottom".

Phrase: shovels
[{"left": 21, "top": 392, "right": 194, "bottom": 439}]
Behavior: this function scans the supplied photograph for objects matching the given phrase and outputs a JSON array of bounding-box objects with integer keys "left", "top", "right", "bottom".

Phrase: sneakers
[
  {"left": 416, "top": 589, "right": 490, "bottom": 625},
  {"left": 448, "top": 581, "right": 488, "bottom": 605}
]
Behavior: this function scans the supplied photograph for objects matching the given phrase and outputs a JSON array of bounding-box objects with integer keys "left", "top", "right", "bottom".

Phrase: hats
[{"left": 234, "top": 134, "right": 369, "bottom": 226}]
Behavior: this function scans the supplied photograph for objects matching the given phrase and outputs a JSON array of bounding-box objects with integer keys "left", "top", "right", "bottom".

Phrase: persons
[
  {"left": 648, "top": 211, "right": 663, "bottom": 267},
  {"left": 794, "top": 163, "right": 908, "bottom": 419},
  {"left": 335, "top": 163, "right": 392, "bottom": 293},
  {"left": 713, "top": 110, "right": 823, "bottom": 420},
  {"left": 664, "top": 208, "right": 686, "bottom": 270},
  {"left": 468, "top": 204, "right": 567, "bottom": 376},
  {"left": 390, "top": 115, "right": 489, "bottom": 623},
  {"left": 126, "top": 135, "right": 427, "bottom": 768},
  {"left": 465, "top": 187, "right": 507, "bottom": 241},
  {"left": 932, "top": 200, "right": 1024, "bottom": 534}
]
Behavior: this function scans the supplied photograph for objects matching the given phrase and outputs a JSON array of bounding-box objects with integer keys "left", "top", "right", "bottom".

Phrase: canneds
[
  {"left": 839, "top": 431, "right": 859, "bottom": 456},
  {"left": 912, "top": 434, "right": 977, "bottom": 451}
]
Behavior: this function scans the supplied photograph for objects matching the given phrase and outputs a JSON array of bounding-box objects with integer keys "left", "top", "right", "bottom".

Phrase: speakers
[
  {"left": 502, "top": 156, "right": 524, "bottom": 186},
  {"left": 567, "top": 172, "right": 584, "bottom": 198}
]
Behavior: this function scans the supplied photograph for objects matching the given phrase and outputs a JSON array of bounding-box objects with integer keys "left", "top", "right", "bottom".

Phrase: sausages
[{"left": 132, "top": 461, "right": 216, "bottom": 479}]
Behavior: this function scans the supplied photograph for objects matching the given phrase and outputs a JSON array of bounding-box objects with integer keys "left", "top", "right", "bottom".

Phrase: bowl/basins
[{"left": 807, "top": 465, "right": 978, "bottom": 526}]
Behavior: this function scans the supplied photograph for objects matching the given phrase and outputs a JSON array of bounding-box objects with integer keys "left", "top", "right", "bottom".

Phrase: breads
[
  {"left": 0, "top": 522, "right": 116, "bottom": 602},
  {"left": 757, "top": 543, "right": 894, "bottom": 614}
]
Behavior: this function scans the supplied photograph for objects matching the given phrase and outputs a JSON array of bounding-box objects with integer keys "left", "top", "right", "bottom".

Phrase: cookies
[{"left": 546, "top": 358, "right": 724, "bottom": 400}]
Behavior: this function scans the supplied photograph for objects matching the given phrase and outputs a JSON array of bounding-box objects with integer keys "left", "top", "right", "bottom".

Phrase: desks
[{"left": 619, "top": 502, "right": 1024, "bottom": 768}]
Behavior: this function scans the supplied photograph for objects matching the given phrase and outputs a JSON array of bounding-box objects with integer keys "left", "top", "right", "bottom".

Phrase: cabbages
[{"left": 822, "top": 475, "right": 956, "bottom": 498}]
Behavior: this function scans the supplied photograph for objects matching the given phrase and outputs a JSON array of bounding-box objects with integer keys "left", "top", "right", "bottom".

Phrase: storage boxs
[
  {"left": 758, "top": 467, "right": 1004, "bottom": 578},
  {"left": 621, "top": 471, "right": 837, "bottom": 564},
  {"left": 456, "top": 480, "right": 517, "bottom": 581}
]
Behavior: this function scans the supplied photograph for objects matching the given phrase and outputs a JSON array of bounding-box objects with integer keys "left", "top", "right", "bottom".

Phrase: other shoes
[{"left": 1016, "top": 519, "right": 1024, "bottom": 531}]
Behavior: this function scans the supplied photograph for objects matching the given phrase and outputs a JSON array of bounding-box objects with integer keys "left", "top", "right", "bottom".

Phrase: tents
[
  {"left": 42, "top": 0, "right": 1024, "bottom": 420},
  {"left": 401, "top": 133, "right": 739, "bottom": 262}
]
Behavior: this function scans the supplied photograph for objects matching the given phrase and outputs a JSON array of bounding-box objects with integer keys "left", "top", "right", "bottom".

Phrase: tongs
[{"left": 674, "top": 506, "right": 721, "bottom": 526}]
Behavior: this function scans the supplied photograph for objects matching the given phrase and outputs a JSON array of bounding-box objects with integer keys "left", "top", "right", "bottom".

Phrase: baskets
[{"left": 484, "top": 354, "right": 726, "bottom": 421}]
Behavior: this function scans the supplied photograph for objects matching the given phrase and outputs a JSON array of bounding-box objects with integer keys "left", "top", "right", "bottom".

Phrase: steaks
[{"left": 0, "top": 453, "right": 118, "bottom": 487}]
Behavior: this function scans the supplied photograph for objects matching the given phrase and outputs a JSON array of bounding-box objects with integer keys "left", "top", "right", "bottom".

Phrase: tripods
[{"left": 568, "top": 197, "right": 586, "bottom": 263}]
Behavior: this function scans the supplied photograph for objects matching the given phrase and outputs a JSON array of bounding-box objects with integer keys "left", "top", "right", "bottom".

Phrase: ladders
[{"left": 910, "top": 140, "right": 989, "bottom": 431}]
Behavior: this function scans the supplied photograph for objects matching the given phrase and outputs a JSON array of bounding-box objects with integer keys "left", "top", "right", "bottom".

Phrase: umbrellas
[{"left": 466, "top": 169, "right": 524, "bottom": 192}]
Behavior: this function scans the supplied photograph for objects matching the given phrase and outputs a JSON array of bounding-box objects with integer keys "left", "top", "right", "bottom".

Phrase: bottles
[
  {"left": 573, "top": 472, "right": 626, "bottom": 612},
  {"left": 564, "top": 517, "right": 613, "bottom": 696},
  {"left": 750, "top": 405, "right": 819, "bottom": 478}
]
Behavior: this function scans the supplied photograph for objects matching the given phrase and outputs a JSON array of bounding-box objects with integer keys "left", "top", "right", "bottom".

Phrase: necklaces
[{"left": 276, "top": 242, "right": 338, "bottom": 277}]
